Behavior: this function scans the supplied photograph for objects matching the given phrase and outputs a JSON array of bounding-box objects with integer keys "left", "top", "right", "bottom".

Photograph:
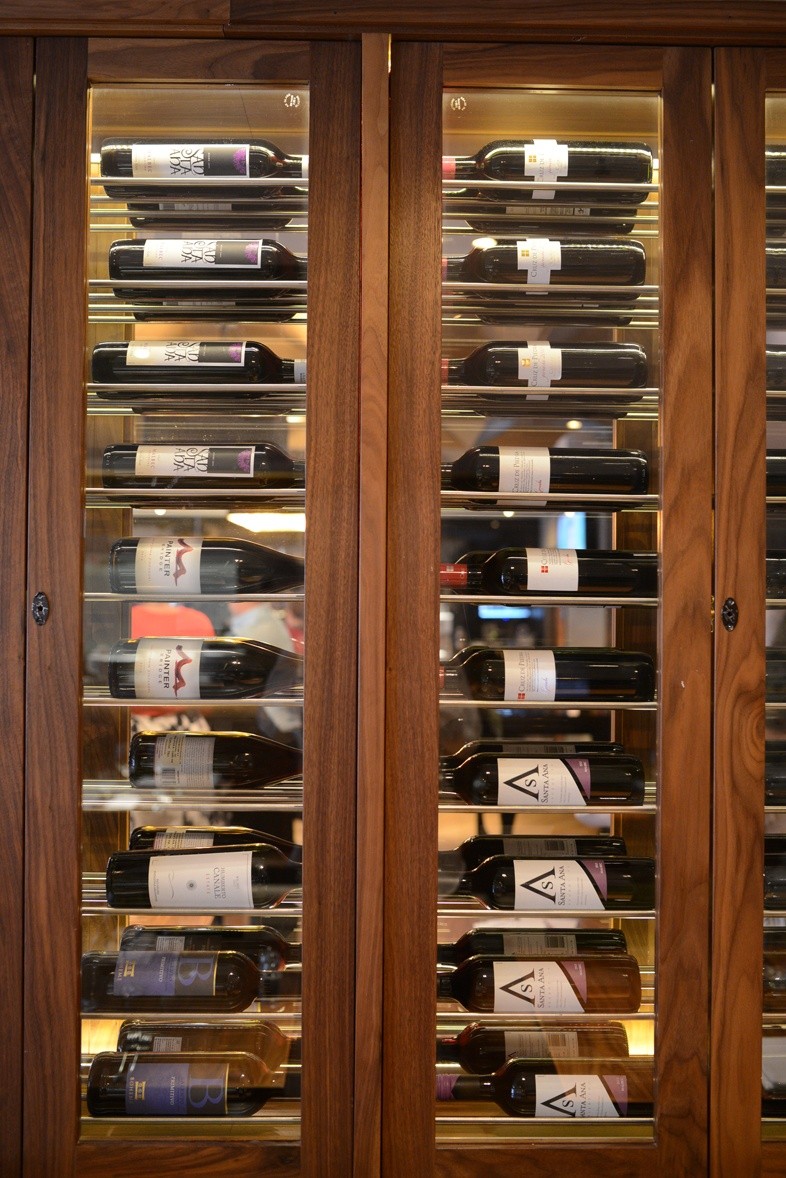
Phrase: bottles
[
  {"left": 437, "top": 646, "right": 654, "bottom": 703},
  {"left": 79, "top": 950, "right": 281, "bottom": 1013},
  {"left": 129, "top": 730, "right": 303, "bottom": 790},
  {"left": 109, "top": 537, "right": 304, "bottom": 592},
  {"left": 130, "top": 824, "right": 303, "bottom": 861},
  {"left": 435, "top": 1019, "right": 656, "bottom": 1118},
  {"left": 437, "top": 954, "right": 642, "bottom": 1013},
  {"left": 442, "top": 339, "right": 648, "bottom": 419},
  {"left": 437, "top": 835, "right": 655, "bottom": 910},
  {"left": 117, "top": 1019, "right": 302, "bottom": 1071},
  {"left": 440, "top": 446, "right": 650, "bottom": 508},
  {"left": 106, "top": 844, "right": 303, "bottom": 910},
  {"left": 100, "top": 138, "right": 310, "bottom": 229},
  {"left": 438, "top": 927, "right": 626, "bottom": 968},
  {"left": 87, "top": 1051, "right": 300, "bottom": 1119},
  {"left": 444, "top": 141, "right": 652, "bottom": 234},
  {"left": 439, "top": 546, "right": 659, "bottom": 598},
  {"left": 438, "top": 740, "right": 645, "bottom": 806},
  {"left": 109, "top": 635, "right": 304, "bottom": 700},
  {"left": 121, "top": 925, "right": 303, "bottom": 973},
  {"left": 442, "top": 235, "right": 646, "bottom": 325},
  {"left": 93, "top": 341, "right": 305, "bottom": 412},
  {"left": 109, "top": 239, "right": 307, "bottom": 322},
  {"left": 102, "top": 442, "right": 306, "bottom": 509}
]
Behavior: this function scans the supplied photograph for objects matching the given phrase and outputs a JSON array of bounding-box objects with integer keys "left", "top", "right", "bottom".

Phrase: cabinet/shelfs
[{"left": 0, "top": 0, "right": 786, "bottom": 1178}]
